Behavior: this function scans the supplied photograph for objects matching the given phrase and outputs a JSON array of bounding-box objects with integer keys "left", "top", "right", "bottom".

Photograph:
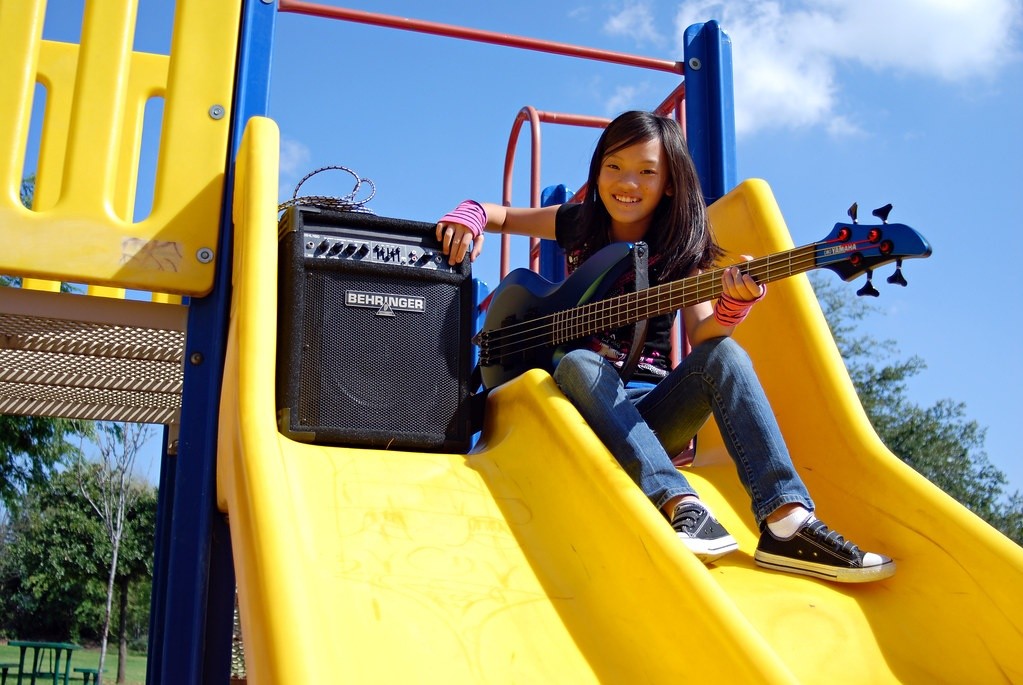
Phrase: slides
[{"left": 205, "top": 105, "right": 1023, "bottom": 685}]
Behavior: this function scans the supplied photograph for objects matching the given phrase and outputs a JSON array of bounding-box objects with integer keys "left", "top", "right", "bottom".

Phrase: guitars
[{"left": 474, "top": 199, "right": 934, "bottom": 391}]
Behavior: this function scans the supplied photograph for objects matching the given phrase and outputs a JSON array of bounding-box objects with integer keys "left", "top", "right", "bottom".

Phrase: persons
[{"left": 435, "top": 111, "right": 896, "bottom": 584}]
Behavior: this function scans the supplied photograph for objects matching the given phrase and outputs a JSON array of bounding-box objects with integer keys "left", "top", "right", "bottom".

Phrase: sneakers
[
  {"left": 668, "top": 500, "right": 739, "bottom": 564},
  {"left": 753, "top": 511, "right": 896, "bottom": 582}
]
